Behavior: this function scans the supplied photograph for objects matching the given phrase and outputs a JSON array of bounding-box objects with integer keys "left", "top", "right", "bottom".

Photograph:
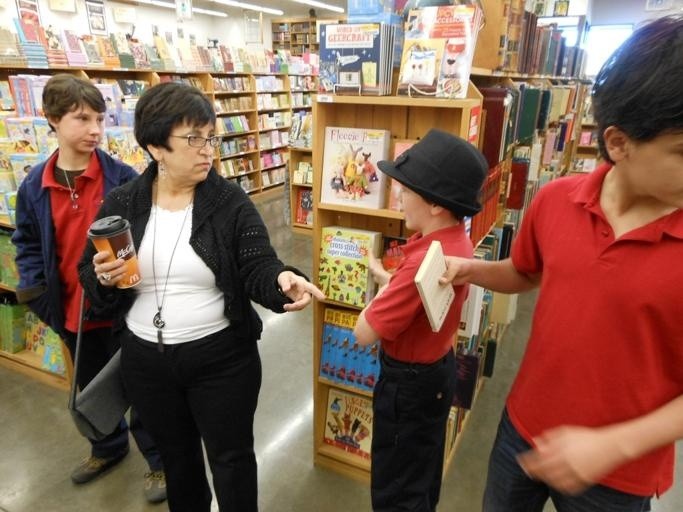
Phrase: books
[
  {"left": 2, "top": 237, "right": 69, "bottom": 377},
  {"left": 0, "top": 13, "right": 325, "bottom": 229},
  {"left": 315, "top": 1, "right": 611, "bottom": 489},
  {"left": 414, "top": 239, "right": 457, "bottom": 333}
]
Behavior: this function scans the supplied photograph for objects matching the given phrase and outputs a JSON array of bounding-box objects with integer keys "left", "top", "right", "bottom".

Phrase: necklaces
[
  {"left": 148, "top": 174, "right": 201, "bottom": 350},
  {"left": 59, "top": 157, "right": 88, "bottom": 214}
]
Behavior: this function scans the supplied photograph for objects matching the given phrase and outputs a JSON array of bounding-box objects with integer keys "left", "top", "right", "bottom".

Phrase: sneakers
[
  {"left": 71, "top": 444, "right": 131, "bottom": 484},
  {"left": 143, "top": 470, "right": 168, "bottom": 504}
]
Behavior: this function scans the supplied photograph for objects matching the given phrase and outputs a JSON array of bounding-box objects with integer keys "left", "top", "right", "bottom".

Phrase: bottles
[{"left": 382, "top": 240, "right": 405, "bottom": 275}]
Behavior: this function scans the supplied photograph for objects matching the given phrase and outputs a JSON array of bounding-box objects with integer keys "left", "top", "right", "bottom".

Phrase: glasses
[{"left": 170, "top": 134, "right": 222, "bottom": 148}]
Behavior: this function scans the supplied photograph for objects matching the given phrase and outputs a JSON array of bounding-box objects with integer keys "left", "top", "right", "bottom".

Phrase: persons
[
  {"left": 434, "top": 10, "right": 683, "bottom": 511},
  {"left": 78, "top": 81, "right": 333, "bottom": 511},
  {"left": 344, "top": 127, "right": 489, "bottom": 511},
  {"left": 12, "top": 71, "right": 171, "bottom": 504}
]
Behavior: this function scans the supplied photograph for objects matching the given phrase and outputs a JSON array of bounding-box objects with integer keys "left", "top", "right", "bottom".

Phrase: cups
[{"left": 86, "top": 215, "right": 142, "bottom": 290}]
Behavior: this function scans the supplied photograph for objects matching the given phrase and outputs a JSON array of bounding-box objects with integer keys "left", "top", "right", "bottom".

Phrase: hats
[{"left": 377, "top": 128, "right": 489, "bottom": 217}]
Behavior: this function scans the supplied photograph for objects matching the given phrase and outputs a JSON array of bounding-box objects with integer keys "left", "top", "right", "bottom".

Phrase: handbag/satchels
[{"left": 68, "top": 347, "right": 132, "bottom": 442}]
